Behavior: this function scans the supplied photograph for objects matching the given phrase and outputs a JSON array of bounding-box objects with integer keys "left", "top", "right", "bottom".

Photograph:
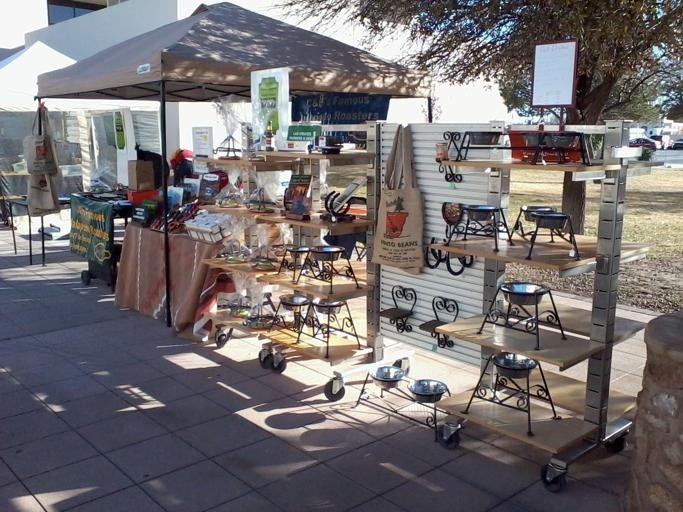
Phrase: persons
[{"left": 170, "top": 149, "right": 195, "bottom": 188}]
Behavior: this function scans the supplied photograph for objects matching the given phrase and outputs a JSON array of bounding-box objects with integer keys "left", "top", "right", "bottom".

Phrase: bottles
[{"left": 266, "top": 121, "right": 274, "bottom": 151}]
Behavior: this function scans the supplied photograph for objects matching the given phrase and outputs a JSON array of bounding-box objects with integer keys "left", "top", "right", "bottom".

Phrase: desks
[
  {"left": 70, "top": 191, "right": 132, "bottom": 294},
  {"left": 6, "top": 194, "right": 71, "bottom": 265},
  {"left": 114, "top": 220, "right": 227, "bottom": 334}
]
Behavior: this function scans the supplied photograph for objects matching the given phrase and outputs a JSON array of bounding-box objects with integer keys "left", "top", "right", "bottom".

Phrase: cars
[
  {"left": 674, "top": 139, "right": 683, "bottom": 150},
  {"left": 629, "top": 137, "right": 661, "bottom": 151}
]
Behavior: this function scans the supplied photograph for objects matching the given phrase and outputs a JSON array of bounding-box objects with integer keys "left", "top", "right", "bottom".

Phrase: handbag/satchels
[
  {"left": 22, "top": 107, "right": 59, "bottom": 175},
  {"left": 370, "top": 126, "right": 421, "bottom": 276},
  {"left": 24, "top": 106, "right": 60, "bottom": 210}
]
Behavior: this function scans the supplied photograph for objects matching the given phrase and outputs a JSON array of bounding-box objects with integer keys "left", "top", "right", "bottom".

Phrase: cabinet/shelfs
[
  {"left": 427, "top": 119, "right": 647, "bottom": 494},
  {"left": 194, "top": 148, "right": 375, "bottom": 404}
]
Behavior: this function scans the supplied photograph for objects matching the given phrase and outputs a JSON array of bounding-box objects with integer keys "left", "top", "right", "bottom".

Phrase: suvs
[{"left": 650, "top": 135, "right": 674, "bottom": 150}]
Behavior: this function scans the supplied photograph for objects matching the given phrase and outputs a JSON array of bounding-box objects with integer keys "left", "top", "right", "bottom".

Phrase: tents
[
  {"left": 35, "top": 3, "right": 433, "bottom": 329},
  {"left": 0, "top": 41, "right": 138, "bottom": 219}
]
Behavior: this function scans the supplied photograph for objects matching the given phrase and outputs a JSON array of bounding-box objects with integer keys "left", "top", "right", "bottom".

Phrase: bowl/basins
[
  {"left": 310, "top": 246, "right": 345, "bottom": 261},
  {"left": 407, "top": 379, "right": 447, "bottom": 403},
  {"left": 279, "top": 295, "right": 309, "bottom": 311},
  {"left": 501, "top": 283, "right": 547, "bottom": 304},
  {"left": 545, "top": 131, "right": 580, "bottom": 148},
  {"left": 493, "top": 352, "right": 537, "bottom": 378},
  {"left": 508, "top": 130, "right": 544, "bottom": 148},
  {"left": 371, "top": 366, "right": 404, "bottom": 388},
  {"left": 534, "top": 212, "right": 568, "bottom": 229},
  {"left": 312, "top": 297, "right": 342, "bottom": 314},
  {"left": 466, "top": 204, "right": 496, "bottom": 221},
  {"left": 467, "top": 131, "right": 502, "bottom": 145},
  {"left": 524, "top": 206, "right": 553, "bottom": 221},
  {"left": 287, "top": 246, "right": 309, "bottom": 259}
]
